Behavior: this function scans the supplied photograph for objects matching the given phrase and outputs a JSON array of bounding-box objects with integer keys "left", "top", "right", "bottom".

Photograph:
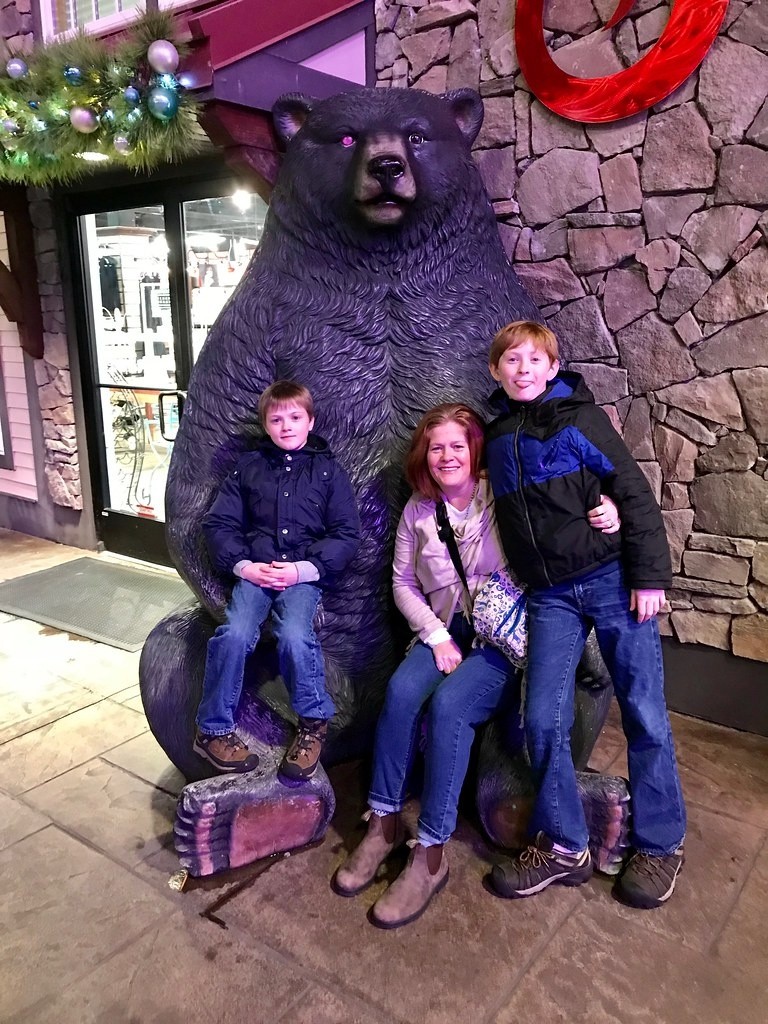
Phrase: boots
[
  {"left": 334, "top": 809, "right": 407, "bottom": 896},
  {"left": 371, "top": 839, "right": 449, "bottom": 929}
]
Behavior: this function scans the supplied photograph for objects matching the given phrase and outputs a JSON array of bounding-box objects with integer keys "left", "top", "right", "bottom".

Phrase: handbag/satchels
[{"left": 435, "top": 497, "right": 529, "bottom": 669}]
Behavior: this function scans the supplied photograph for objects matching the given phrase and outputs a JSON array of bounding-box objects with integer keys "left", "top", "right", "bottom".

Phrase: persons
[
  {"left": 189, "top": 381, "right": 360, "bottom": 781},
  {"left": 202, "top": 266, "right": 219, "bottom": 288},
  {"left": 476, "top": 319, "right": 689, "bottom": 909},
  {"left": 330, "top": 402, "right": 625, "bottom": 930}
]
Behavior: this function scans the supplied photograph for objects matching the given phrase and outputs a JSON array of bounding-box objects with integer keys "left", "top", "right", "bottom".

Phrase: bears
[{"left": 138, "top": 88, "right": 636, "bottom": 879}]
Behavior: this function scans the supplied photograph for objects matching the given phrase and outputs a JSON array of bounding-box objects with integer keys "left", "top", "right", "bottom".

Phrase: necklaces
[{"left": 444, "top": 479, "right": 479, "bottom": 539}]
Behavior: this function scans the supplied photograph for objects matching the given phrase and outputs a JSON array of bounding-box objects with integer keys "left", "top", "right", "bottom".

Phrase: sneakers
[
  {"left": 491, "top": 831, "right": 593, "bottom": 899},
  {"left": 193, "top": 729, "right": 259, "bottom": 773},
  {"left": 621, "top": 840, "right": 685, "bottom": 909},
  {"left": 278, "top": 715, "right": 328, "bottom": 781}
]
